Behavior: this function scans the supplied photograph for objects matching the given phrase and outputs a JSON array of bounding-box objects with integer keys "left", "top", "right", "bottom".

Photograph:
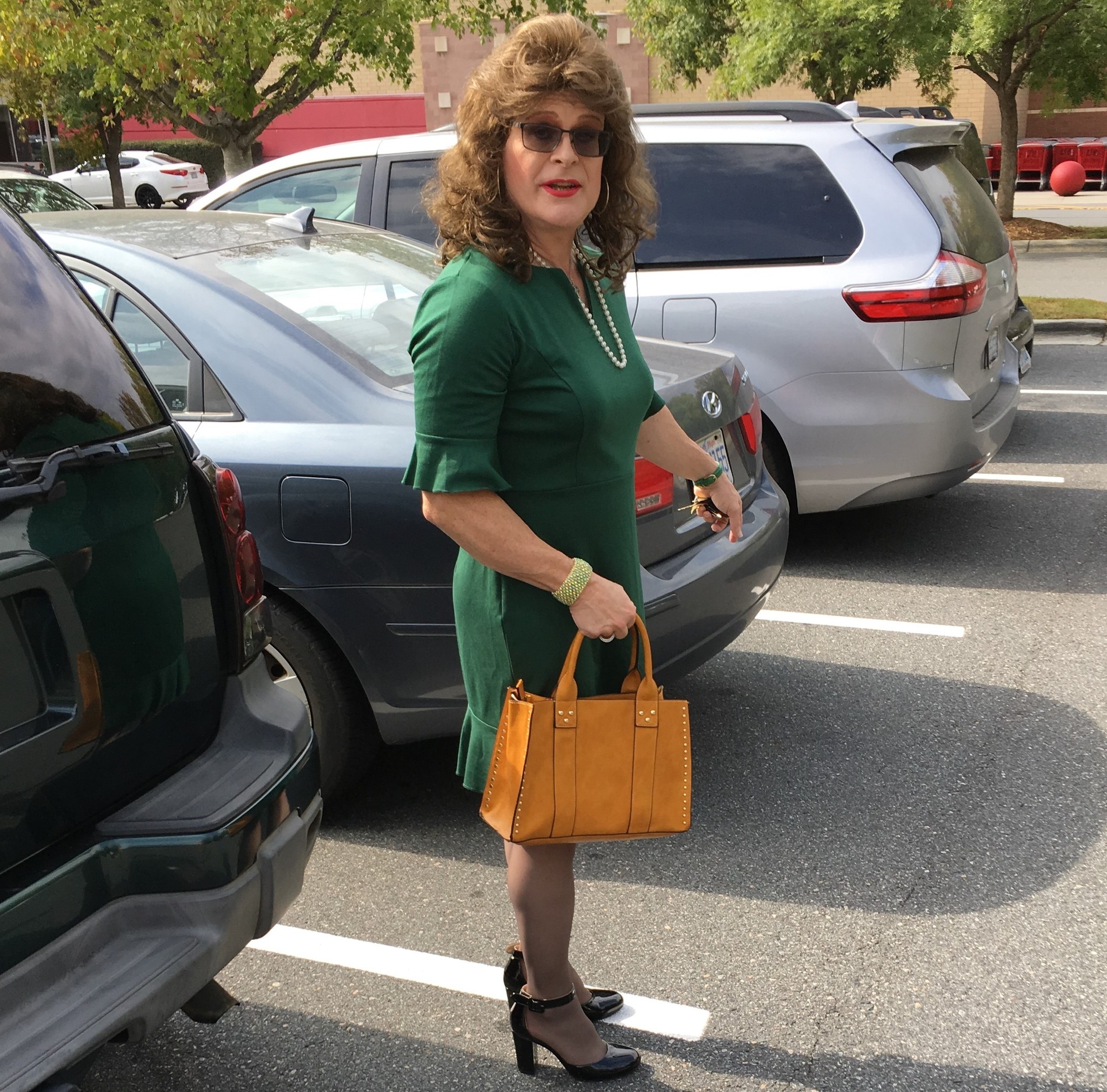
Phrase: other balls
[{"left": 1049, "top": 161, "right": 1086, "bottom": 196}]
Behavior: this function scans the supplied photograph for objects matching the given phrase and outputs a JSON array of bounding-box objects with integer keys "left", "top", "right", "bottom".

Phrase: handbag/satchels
[{"left": 479, "top": 611, "right": 691, "bottom": 845}]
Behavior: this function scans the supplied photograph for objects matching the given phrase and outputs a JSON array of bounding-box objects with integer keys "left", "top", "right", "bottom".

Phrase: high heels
[
  {"left": 503, "top": 943, "right": 623, "bottom": 1021},
  {"left": 510, "top": 983, "right": 641, "bottom": 1081}
]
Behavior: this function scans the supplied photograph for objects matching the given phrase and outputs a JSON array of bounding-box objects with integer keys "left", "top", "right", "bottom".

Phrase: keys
[{"left": 677, "top": 498, "right": 728, "bottom": 518}]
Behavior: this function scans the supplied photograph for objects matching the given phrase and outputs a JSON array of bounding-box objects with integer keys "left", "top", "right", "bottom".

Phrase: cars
[
  {"left": 47, "top": 149, "right": 211, "bottom": 209},
  {"left": 15, "top": 209, "right": 790, "bottom": 798},
  {"left": 0, "top": 162, "right": 100, "bottom": 215}
]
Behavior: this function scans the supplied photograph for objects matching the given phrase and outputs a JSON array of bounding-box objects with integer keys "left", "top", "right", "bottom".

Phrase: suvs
[
  {"left": 185, "top": 97, "right": 1042, "bottom": 537},
  {"left": 0, "top": 196, "right": 326, "bottom": 1092}
]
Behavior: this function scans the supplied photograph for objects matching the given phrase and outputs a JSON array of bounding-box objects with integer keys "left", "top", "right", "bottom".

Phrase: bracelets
[
  {"left": 694, "top": 462, "right": 723, "bottom": 488},
  {"left": 552, "top": 557, "right": 593, "bottom": 606}
]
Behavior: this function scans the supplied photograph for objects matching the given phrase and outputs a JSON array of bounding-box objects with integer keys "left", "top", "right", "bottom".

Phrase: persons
[{"left": 402, "top": 12, "right": 743, "bottom": 1081}]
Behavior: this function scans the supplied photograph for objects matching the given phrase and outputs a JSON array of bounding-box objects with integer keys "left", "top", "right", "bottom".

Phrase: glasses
[{"left": 513, "top": 120, "right": 614, "bottom": 157}]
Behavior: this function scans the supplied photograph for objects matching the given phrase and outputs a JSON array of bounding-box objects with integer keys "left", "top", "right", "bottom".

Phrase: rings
[{"left": 599, "top": 635, "right": 615, "bottom": 642}]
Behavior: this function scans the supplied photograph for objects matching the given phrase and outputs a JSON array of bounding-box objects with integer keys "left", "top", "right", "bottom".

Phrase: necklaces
[{"left": 527, "top": 239, "right": 627, "bottom": 369}]
